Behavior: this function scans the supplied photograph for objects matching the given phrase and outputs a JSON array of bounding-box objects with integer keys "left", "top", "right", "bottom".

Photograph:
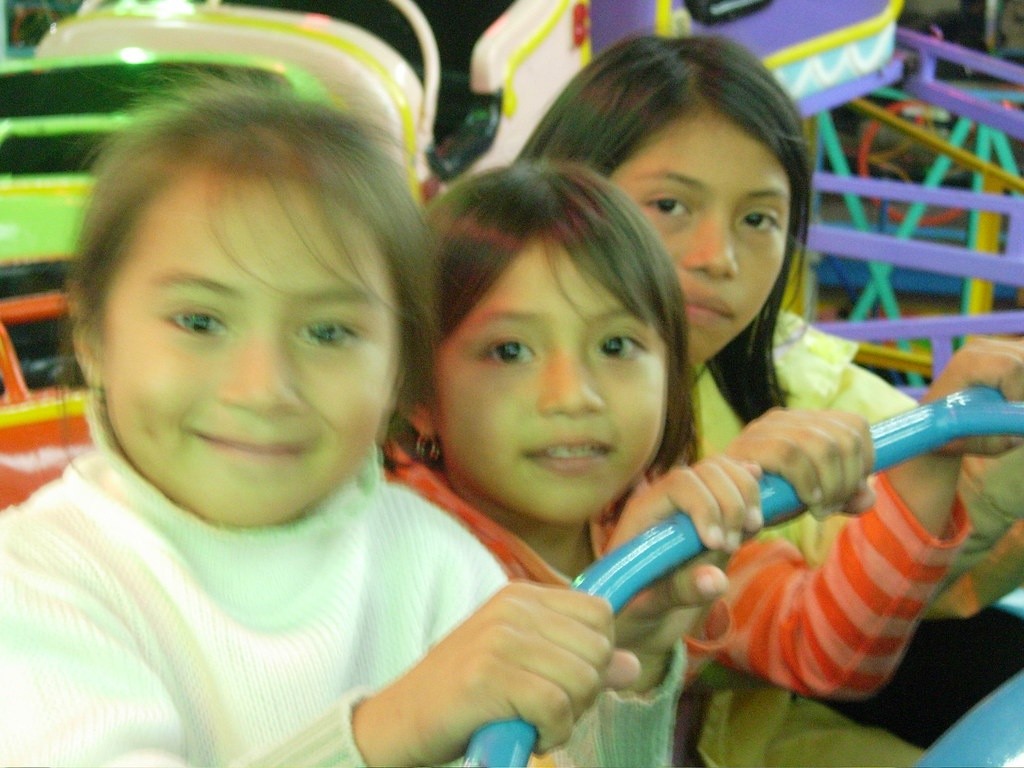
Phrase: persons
[
  {"left": 383, "top": 33, "right": 1024, "bottom": 768},
  {"left": 0, "top": 61, "right": 763, "bottom": 768}
]
